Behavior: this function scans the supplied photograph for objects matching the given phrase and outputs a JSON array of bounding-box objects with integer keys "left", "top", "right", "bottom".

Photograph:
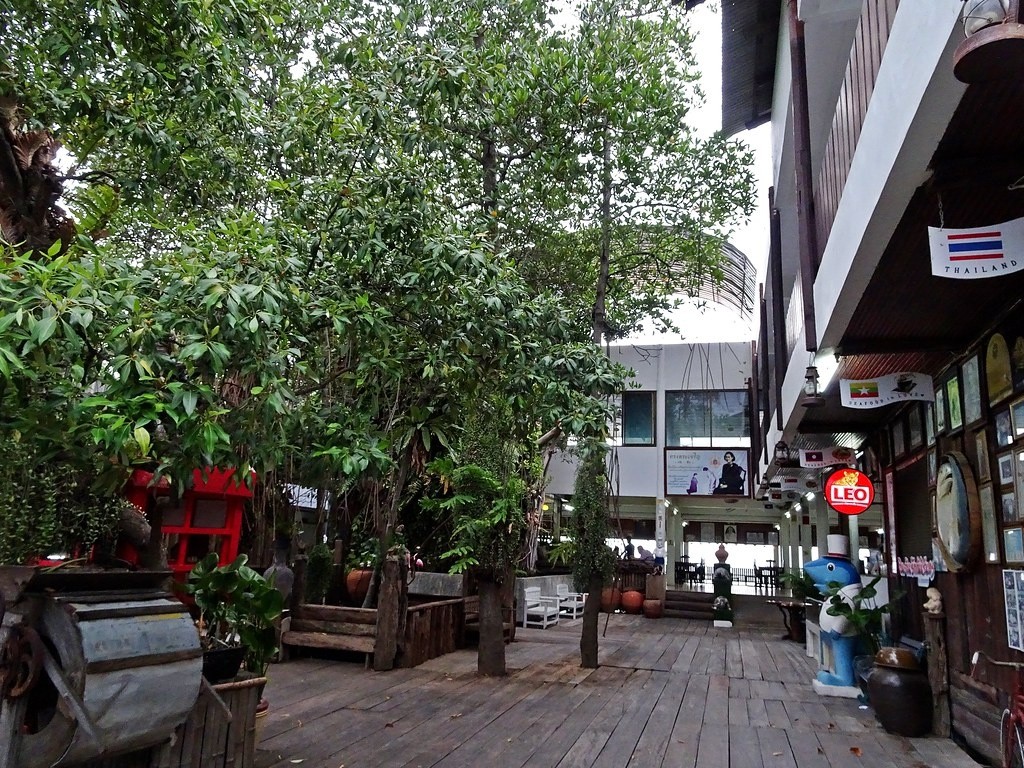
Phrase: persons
[
  {"left": 637, "top": 546, "right": 652, "bottom": 558},
  {"left": 713, "top": 452, "right": 746, "bottom": 494},
  {"left": 703, "top": 467, "right": 716, "bottom": 492},
  {"left": 625, "top": 535, "right": 634, "bottom": 559},
  {"left": 687, "top": 473, "right": 698, "bottom": 495}
]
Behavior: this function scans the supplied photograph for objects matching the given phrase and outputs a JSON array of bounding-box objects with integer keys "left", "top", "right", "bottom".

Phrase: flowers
[
  {"left": 344, "top": 537, "right": 380, "bottom": 568},
  {"left": 711, "top": 568, "right": 734, "bottom": 586},
  {"left": 710, "top": 596, "right": 732, "bottom": 611}
]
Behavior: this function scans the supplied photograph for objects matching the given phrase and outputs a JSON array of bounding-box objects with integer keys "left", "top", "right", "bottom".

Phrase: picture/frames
[
  {"left": 995, "top": 449, "right": 1013, "bottom": 489},
  {"left": 889, "top": 411, "right": 909, "bottom": 466},
  {"left": 990, "top": 406, "right": 1017, "bottom": 453},
  {"left": 958, "top": 346, "right": 989, "bottom": 435},
  {"left": 1009, "top": 395, "right": 1024, "bottom": 440},
  {"left": 943, "top": 371, "right": 965, "bottom": 439},
  {"left": 1011, "top": 443, "right": 1024, "bottom": 522},
  {"left": 933, "top": 385, "right": 947, "bottom": 437},
  {"left": 924, "top": 401, "right": 937, "bottom": 450},
  {"left": 927, "top": 446, "right": 939, "bottom": 488},
  {"left": 977, "top": 481, "right": 1006, "bottom": 565},
  {"left": 1001, "top": 525, "right": 1024, "bottom": 567},
  {"left": 905, "top": 403, "right": 926, "bottom": 456}
]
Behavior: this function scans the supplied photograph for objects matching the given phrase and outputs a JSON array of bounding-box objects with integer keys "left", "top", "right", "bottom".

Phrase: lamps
[
  {"left": 800, "top": 352, "right": 826, "bottom": 409},
  {"left": 793, "top": 502, "right": 802, "bottom": 512},
  {"left": 774, "top": 523, "right": 781, "bottom": 531},
  {"left": 772, "top": 430, "right": 791, "bottom": 466},
  {"left": 785, "top": 511, "right": 791, "bottom": 519},
  {"left": 672, "top": 506, "right": 679, "bottom": 515},
  {"left": 759, "top": 473, "right": 770, "bottom": 489},
  {"left": 681, "top": 520, "right": 689, "bottom": 527},
  {"left": 805, "top": 491, "right": 816, "bottom": 502},
  {"left": 665, "top": 498, "right": 670, "bottom": 507}
]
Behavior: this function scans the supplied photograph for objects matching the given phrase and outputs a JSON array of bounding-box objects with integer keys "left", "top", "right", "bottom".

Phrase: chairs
[
  {"left": 556, "top": 584, "right": 589, "bottom": 620},
  {"left": 771, "top": 559, "right": 785, "bottom": 586},
  {"left": 523, "top": 586, "right": 565, "bottom": 630},
  {"left": 752, "top": 559, "right": 767, "bottom": 586},
  {"left": 675, "top": 557, "right": 706, "bottom": 583}
]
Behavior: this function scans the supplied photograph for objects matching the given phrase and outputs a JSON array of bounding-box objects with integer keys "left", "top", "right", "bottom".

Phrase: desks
[
  {"left": 758, "top": 567, "right": 779, "bottom": 586},
  {"left": 675, "top": 562, "right": 699, "bottom": 583}
]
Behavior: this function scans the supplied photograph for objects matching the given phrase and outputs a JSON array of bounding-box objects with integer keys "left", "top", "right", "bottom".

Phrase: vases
[
  {"left": 346, "top": 567, "right": 373, "bottom": 599},
  {"left": 714, "top": 582, "right": 731, "bottom": 594},
  {"left": 712, "top": 609, "right": 731, "bottom": 621},
  {"left": 643, "top": 599, "right": 663, "bottom": 619}
]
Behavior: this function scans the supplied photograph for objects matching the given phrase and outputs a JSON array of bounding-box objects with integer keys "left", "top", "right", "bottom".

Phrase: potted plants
[
  {"left": 182, "top": 554, "right": 285, "bottom": 682},
  {"left": 236, "top": 564, "right": 281, "bottom": 705}
]
